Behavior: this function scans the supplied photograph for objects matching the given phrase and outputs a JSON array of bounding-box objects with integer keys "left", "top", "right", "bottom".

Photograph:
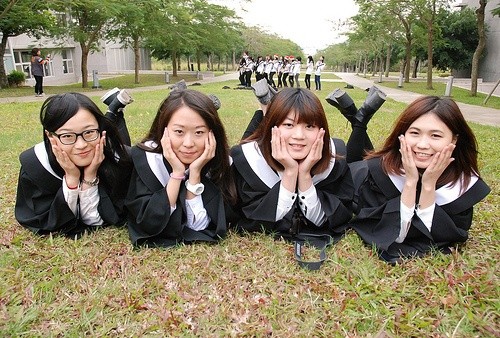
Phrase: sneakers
[
  {"left": 101, "top": 87, "right": 120, "bottom": 106},
  {"left": 108, "top": 89, "right": 135, "bottom": 116}
]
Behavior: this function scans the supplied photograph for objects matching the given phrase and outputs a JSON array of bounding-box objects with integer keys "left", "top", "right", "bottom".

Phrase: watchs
[
  {"left": 185, "top": 180, "right": 205, "bottom": 195},
  {"left": 81, "top": 178, "right": 100, "bottom": 187}
]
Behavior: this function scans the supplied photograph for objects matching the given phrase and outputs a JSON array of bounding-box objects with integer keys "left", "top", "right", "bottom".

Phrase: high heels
[
  {"left": 325, "top": 88, "right": 359, "bottom": 121},
  {"left": 354, "top": 86, "right": 387, "bottom": 126}
]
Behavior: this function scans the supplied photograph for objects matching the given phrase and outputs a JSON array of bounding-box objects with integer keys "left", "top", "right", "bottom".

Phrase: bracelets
[
  {"left": 169, "top": 173, "right": 186, "bottom": 180},
  {"left": 65, "top": 182, "right": 80, "bottom": 190}
]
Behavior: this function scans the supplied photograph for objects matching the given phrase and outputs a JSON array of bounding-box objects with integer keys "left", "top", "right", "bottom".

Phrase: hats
[
  {"left": 289, "top": 55, "right": 294, "bottom": 61},
  {"left": 285, "top": 56, "right": 289, "bottom": 61}
]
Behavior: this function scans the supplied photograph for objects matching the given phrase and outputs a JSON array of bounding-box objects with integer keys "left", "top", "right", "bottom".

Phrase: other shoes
[
  {"left": 267, "top": 82, "right": 279, "bottom": 96},
  {"left": 251, "top": 78, "right": 271, "bottom": 105},
  {"left": 39, "top": 93, "right": 45, "bottom": 97},
  {"left": 34, "top": 92, "right": 39, "bottom": 97},
  {"left": 206, "top": 94, "right": 222, "bottom": 110}
]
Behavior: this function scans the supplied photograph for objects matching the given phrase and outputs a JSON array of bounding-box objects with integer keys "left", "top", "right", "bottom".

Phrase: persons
[
  {"left": 230, "top": 78, "right": 354, "bottom": 249},
  {"left": 325, "top": 84, "right": 492, "bottom": 267},
  {"left": 126, "top": 79, "right": 237, "bottom": 252},
  {"left": 31, "top": 48, "right": 50, "bottom": 97},
  {"left": 236, "top": 51, "right": 325, "bottom": 91},
  {"left": 15, "top": 87, "right": 134, "bottom": 240}
]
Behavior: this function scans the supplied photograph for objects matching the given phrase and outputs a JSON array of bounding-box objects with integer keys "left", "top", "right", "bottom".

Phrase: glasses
[{"left": 50, "top": 128, "right": 100, "bottom": 145}]
